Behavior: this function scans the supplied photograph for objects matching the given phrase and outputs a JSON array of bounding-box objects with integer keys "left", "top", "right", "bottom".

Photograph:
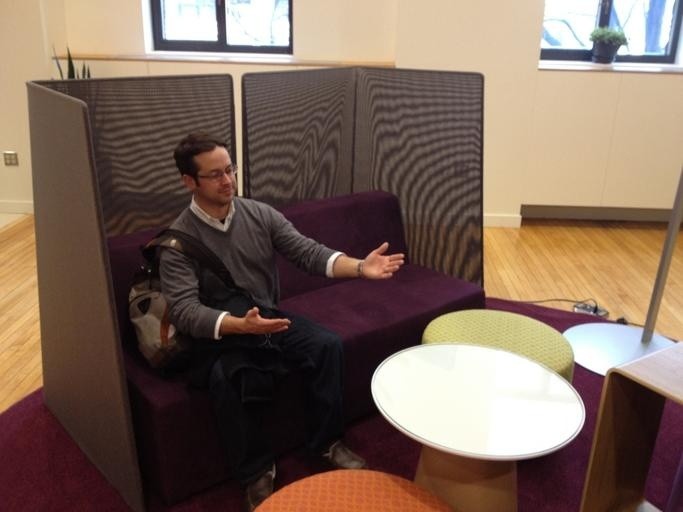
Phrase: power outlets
[{"left": 3, "top": 151, "right": 18, "bottom": 165}]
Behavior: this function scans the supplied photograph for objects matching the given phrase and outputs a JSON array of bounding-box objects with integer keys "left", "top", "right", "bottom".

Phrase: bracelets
[{"left": 355, "top": 256, "right": 369, "bottom": 276}]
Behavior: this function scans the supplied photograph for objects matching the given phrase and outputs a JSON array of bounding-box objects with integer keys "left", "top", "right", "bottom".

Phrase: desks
[{"left": 371, "top": 342, "right": 587, "bottom": 512}]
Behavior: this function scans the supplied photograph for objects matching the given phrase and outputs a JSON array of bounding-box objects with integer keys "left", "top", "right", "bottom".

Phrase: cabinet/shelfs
[{"left": 579, "top": 341, "right": 682, "bottom": 512}]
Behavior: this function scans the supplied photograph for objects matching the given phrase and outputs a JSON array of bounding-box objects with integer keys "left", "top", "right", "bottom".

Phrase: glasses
[{"left": 195, "top": 165, "right": 236, "bottom": 181}]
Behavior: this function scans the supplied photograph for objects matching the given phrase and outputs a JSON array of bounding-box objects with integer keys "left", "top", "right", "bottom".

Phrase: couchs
[{"left": 108, "top": 190, "right": 486, "bottom": 506}]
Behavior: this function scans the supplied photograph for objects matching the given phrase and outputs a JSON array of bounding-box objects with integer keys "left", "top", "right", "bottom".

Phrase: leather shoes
[
  {"left": 244, "top": 460, "right": 279, "bottom": 512},
  {"left": 319, "top": 441, "right": 377, "bottom": 469}
]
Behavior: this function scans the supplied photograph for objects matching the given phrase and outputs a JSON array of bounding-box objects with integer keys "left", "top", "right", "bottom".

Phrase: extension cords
[{"left": 574, "top": 302, "right": 609, "bottom": 318}]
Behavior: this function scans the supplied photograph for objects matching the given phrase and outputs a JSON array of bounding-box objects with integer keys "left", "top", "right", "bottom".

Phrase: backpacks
[{"left": 127, "top": 229, "right": 251, "bottom": 379}]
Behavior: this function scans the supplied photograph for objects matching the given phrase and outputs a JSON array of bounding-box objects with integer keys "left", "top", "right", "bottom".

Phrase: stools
[
  {"left": 251, "top": 468, "right": 456, "bottom": 512},
  {"left": 422, "top": 308, "right": 574, "bottom": 386}
]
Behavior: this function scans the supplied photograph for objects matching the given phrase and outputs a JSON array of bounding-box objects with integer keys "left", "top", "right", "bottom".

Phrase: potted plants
[{"left": 588, "top": 26, "right": 626, "bottom": 65}]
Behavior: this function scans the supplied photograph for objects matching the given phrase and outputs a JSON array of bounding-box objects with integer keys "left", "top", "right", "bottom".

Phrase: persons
[{"left": 164, "top": 127, "right": 407, "bottom": 509}]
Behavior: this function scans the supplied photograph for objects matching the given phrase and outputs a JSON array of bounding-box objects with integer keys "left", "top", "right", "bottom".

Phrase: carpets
[{"left": 1, "top": 298, "right": 681, "bottom": 511}]
